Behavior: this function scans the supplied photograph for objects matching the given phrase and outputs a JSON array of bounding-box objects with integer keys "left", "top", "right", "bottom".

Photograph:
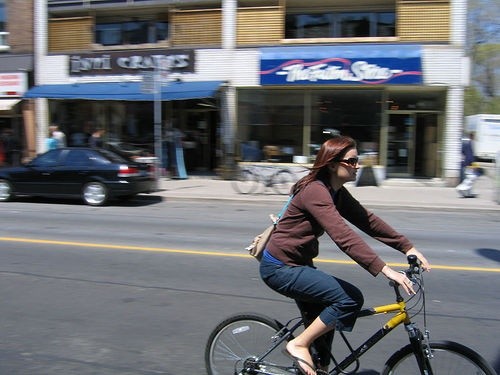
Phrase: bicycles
[
  {"left": 205, "top": 253, "right": 498, "bottom": 375},
  {"left": 232, "top": 158, "right": 298, "bottom": 195}
]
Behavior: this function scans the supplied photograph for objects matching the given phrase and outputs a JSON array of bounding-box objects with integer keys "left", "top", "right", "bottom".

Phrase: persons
[
  {"left": 260, "top": 136, "right": 429, "bottom": 375},
  {"left": 89, "top": 124, "right": 105, "bottom": 148},
  {"left": 46, "top": 125, "right": 66, "bottom": 151},
  {"left": 456, "top": 133, "right": 478, "bottom": 197}
]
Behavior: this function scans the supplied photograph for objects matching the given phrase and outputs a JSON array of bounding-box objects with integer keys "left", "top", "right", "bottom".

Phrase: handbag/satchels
[{"left": 245, "top": 192, "right": 295, "bottom": 263}]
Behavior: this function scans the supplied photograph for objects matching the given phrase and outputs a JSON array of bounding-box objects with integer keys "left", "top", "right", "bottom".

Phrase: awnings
[
  {"left": 0, "top": 99, "right": 21, "bottom": 110},
  {"left": 23, "top": 80, "right": 228, "bottom": 99}
]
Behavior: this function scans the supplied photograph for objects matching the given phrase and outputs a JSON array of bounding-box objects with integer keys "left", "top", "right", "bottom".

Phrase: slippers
[{"left": 281, "top": 348, "right": 317, "bottom": 375}]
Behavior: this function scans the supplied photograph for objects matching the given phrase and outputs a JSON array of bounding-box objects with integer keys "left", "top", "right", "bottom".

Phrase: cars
[{"left": 1, "top": 143, "right": 162, "bottom": 208}]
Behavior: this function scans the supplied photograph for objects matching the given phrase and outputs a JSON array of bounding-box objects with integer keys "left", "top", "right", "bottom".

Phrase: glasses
[{"left": 335, "top": 157, "right": 358, "bottom": 166}]
